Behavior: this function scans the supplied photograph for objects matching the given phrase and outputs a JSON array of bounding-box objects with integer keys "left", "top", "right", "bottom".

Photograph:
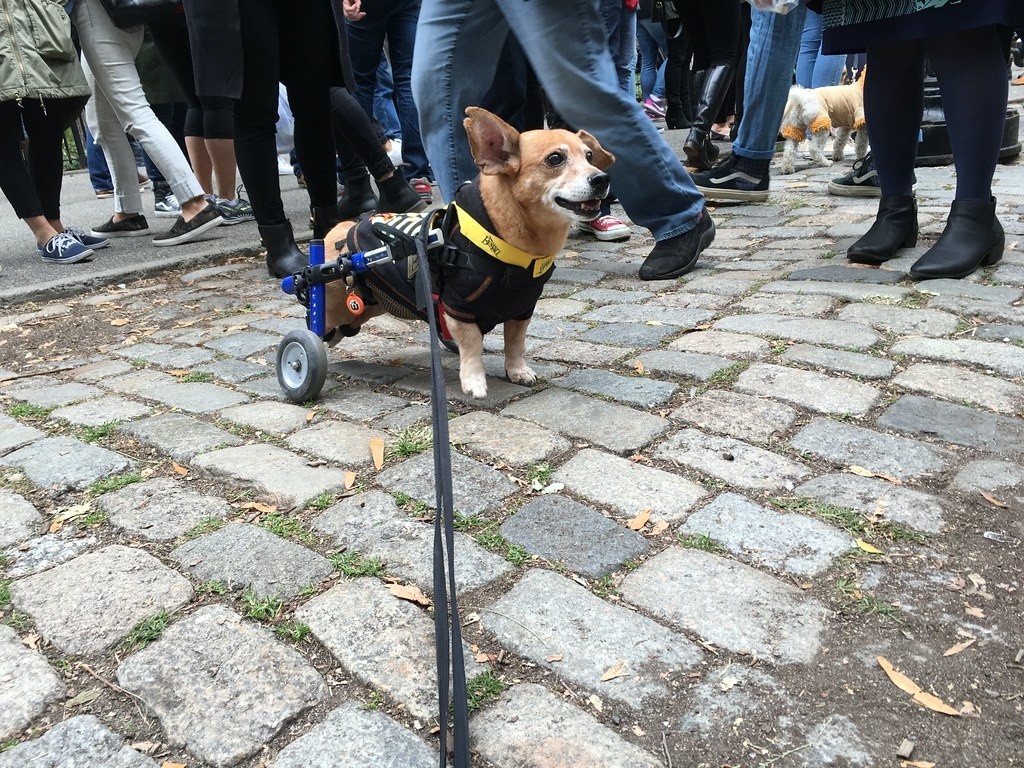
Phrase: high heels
[{"left": 664, "top": 107, "right": 693, "bottom": 129}]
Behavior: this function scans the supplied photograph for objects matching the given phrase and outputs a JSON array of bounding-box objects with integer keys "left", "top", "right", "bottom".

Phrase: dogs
[
  {"left": 779, "top": 62, "right": 870, "bottom": 175},
  {"left": 324, "top": 104, "right": 618, "bottom": 398}
]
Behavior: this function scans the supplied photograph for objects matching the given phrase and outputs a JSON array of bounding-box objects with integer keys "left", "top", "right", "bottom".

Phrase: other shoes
[
  {"left": 296, "top": 174, "right": 307, "bottom": 188},
  {"left": 708, "top": 122, "right": 736, "bottom": 140},
  {"left": 95, "top": 174, "right": 150, "bottom": 199}
]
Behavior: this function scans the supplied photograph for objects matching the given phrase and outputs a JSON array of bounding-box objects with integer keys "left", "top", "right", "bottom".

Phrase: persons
[{"left": 1, "top": 0, "right": 1024, "bottom": 278}]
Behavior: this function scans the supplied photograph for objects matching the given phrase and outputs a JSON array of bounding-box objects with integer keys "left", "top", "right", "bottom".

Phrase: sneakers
[
  {"left": 276, "top": 155, "right": 295, "bottom": 175},
  {"left": 153, "top": 201, "right": 223, "bottom": 246},
  {"left": 643, "top": 93, "right": 668, "bottom": 122},
  {"left": 828, "top": 151, "right": 918, "bottom": 197},
  {"left": 685, "top": 151, "right": 770, "bottom": 201},
  {"left": 37, "top": 226, "right": 110, "bottom": 264},
  {"left": 206, "top": 183, "right": 257, "bottom": 225},
  {"left": 155, "top": 194, "right": 183, "bottom": 219},
  {"left": 578, "top": 215, "right": 632, "bottom": 242},
  {"left": 638, "top": 207, "right": 716, "bottom": 280},
  {"left": 90, "top": 215, "right": 151, "bottom": 238},
  {"left": 335, "top": 139, "right": 433, "bottom": 223}
]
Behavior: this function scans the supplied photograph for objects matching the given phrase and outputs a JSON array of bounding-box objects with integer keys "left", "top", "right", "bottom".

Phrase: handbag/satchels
[
  {"left": 272, "top": 83, "right": 296, "bottom": 155},
  {"left": 746, "top": 0, "right": 801, "bottom": 15},
  {"left": 100, "top": 0, "right": 163, "bottom": 29}
]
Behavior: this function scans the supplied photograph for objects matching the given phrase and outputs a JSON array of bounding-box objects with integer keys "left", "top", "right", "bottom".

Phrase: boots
[
  {"left": 683, "top": 56, "right": 739, "bottom": 170},
  {"left": 846, "top": 197, "right": 919, "bottom": 262},
  {"left": 259, "top": 218, "right": 310, "bottom": 279},
  {"left": 908, "top": 196, "right": 1006, "bottom": 278}
]
